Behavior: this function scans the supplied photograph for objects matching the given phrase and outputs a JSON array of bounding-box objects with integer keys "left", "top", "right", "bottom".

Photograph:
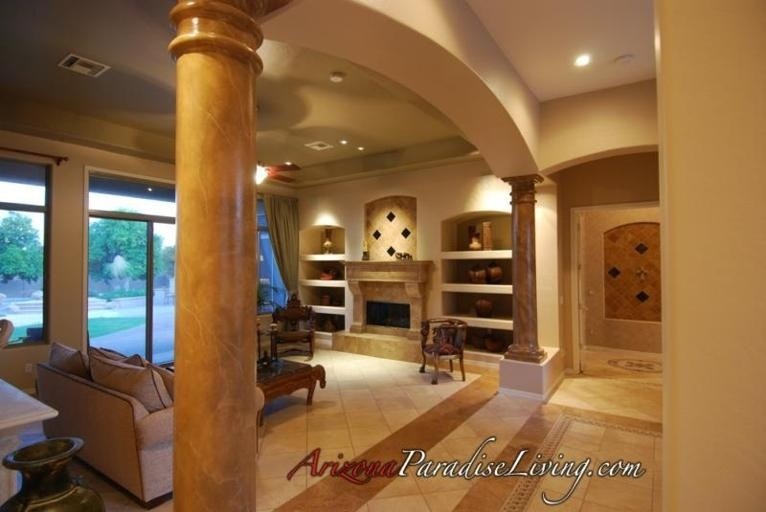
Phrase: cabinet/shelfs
[
  {"left": 440, "top": 250, "right": 516, "bottom": 330},
  {"left": 300, "top": 251, "right": 346, "bottom": 316}
]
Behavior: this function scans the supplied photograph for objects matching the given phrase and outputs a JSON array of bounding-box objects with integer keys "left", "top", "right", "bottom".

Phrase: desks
[
  {"left": 1, "top": 376, "right": 58, "bottom": 506},
  {"left": 254, "top": 356, "right": 326, "bottom": 427}
]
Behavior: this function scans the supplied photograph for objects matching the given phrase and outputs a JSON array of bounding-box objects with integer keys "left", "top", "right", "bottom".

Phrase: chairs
[
  {"left": 267, "top": 293, "right": 318, "bottom": 360},
  {"left": 417, "top": 317, "right": 467, "bottom": 385}
]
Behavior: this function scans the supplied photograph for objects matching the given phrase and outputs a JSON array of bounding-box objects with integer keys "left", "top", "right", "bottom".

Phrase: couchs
[{"left": 34, "top": 360, "right": 192, "bottom": 510}]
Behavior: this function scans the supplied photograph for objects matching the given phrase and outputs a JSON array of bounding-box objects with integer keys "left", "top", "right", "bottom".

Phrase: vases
[{"left": 2, "top": 433, "right": 109, "bottom": 512}]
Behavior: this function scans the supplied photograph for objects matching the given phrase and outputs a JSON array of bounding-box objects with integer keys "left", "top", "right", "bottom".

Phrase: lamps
[{"left": 312, "top": 213, "right": 345, "bottom": 250}]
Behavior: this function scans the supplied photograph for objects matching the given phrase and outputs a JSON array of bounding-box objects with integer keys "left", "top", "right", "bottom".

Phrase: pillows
[{"left": 50, "top": 338, "right": 179, "bottom": 416}]
